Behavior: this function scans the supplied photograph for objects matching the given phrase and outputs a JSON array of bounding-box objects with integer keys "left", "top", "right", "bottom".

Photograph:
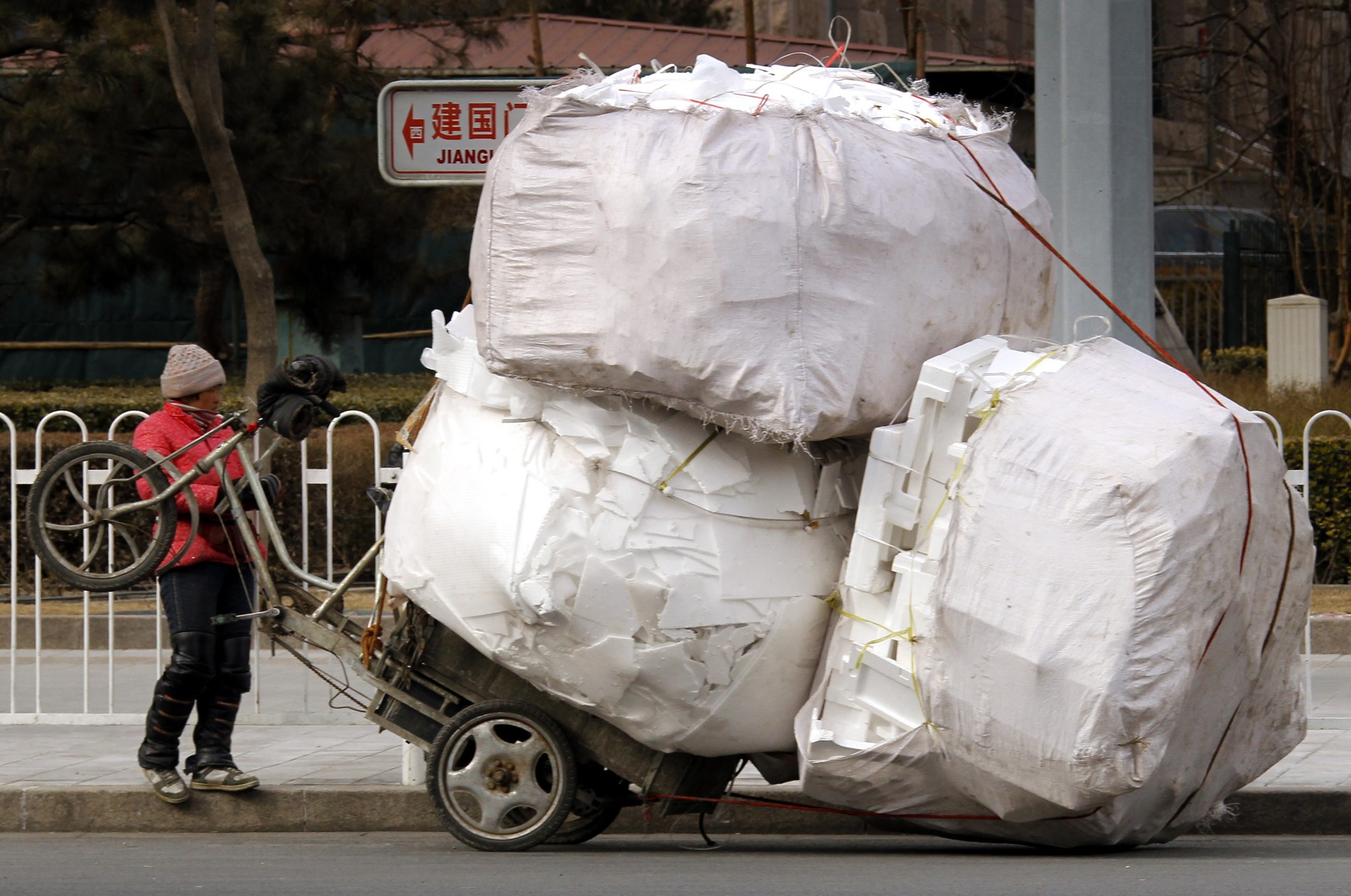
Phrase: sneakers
[
  {"left": 191, "top": 765, "right": 259, "bottom": 790},
  {"left": 143, "top": 767, "right": 188, "bottom": 803}
]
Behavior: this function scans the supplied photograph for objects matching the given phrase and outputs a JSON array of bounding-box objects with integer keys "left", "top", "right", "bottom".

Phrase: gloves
[
  {"left": 217, "top": 477, "right": 258, "bottom": 512},
  {"left": 257, "top": 473, "right": 284, "bottom": 507}
]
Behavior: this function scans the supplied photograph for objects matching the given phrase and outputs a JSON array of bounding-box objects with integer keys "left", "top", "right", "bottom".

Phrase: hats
[{"left": 160, "top": 344, "right": 227, "bottom": 398}]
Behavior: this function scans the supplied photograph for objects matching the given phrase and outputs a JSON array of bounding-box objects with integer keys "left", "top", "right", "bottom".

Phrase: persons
[{"left": 133, "top": 345, "right": 282, "bottom": 803}]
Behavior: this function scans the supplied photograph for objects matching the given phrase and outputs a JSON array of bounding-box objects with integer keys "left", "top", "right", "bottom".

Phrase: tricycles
[{"left": 26, "top": 353, "right": 749, "bottom": 853}]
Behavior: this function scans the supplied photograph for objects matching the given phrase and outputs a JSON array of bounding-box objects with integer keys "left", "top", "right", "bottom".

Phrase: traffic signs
[{"left": 378, "top": 77, "right": 562, "bottom": 189}]
